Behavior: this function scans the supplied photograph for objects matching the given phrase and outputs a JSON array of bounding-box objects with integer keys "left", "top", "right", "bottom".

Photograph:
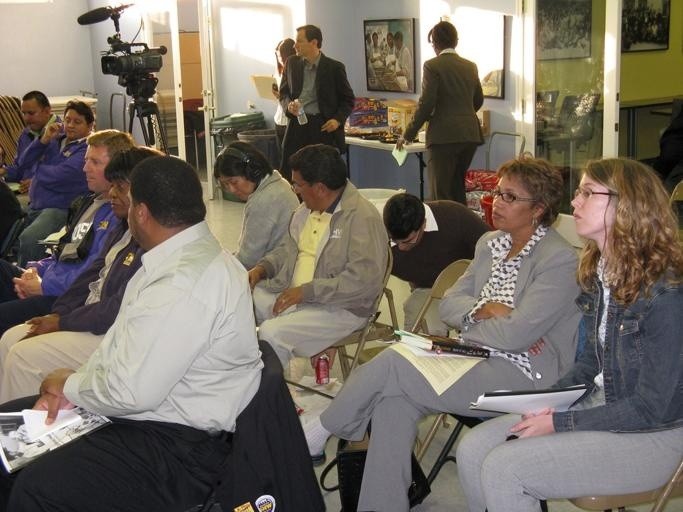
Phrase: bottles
[{"left": 294, "top": 99, "right": 308, "bottom": 126}]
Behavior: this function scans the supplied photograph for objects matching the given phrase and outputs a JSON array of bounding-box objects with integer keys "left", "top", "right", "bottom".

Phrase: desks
[{"left": 343, "top": 135, "right": 428, "bottom": 202}]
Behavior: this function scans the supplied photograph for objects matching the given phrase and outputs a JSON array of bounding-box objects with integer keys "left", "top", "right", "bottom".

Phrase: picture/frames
[
  {"left": 535, "top": 1, "right": 591, "bottom": 60},
  {"left": 451, "top": 15, "right": 506, "bottom": 99},
  {"left": 364, "top": 19, "right": 418, "bottom": 95},
  {"left": 623, "top": 0, "right": 670, "bottom": 53}
]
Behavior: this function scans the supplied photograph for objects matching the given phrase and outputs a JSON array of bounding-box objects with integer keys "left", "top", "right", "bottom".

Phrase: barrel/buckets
[
  {"left": 357, "top": 187, "right": 406, "bottom": 329},
  {"left": 235, "top": 129, "right": 278, "bottom": 164},
  {"left": 476, "top": 195, "right": 495, "bottom": 228}
]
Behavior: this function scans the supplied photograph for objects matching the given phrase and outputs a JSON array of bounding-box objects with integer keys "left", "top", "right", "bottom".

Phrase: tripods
[{"left": 118, "top": 101, "right": 169, "bottom": 157}]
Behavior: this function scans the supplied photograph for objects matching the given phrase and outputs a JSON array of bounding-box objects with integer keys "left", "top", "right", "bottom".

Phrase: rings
[{"left": 282, "top": 298, "right": 285, "bottom": 304}]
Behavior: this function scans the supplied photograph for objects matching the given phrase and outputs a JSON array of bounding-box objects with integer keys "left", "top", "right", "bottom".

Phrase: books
[
  {"left": 399, "top": 334, "right": 491, "bottom": 359},
  {"left": 469, "top": 384, "right": 588, "bottom": 416}
]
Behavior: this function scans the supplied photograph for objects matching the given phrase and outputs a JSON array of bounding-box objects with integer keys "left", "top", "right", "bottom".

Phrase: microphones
[{"left": 77, "top": 7, "right": 111, "bottom": 25}]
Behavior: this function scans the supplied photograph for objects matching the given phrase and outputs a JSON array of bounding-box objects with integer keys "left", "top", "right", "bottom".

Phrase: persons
[
  {"left": 1, "top": 88, "right": 165, "bottom": 402},
  {"left": 1, "top": 156, "right": 265, "bottom": 512},
  {"left": 251, "top": 144, "right": 390, "bottom": 376},
  {"left": 278, "top": 25, "right": 355, "bottom": 204},
  {"left": 308, "top": 192, "right": 493, "bottom": 368},
  {"left": 272, "top": 37, "right": 296, "bottom": 163},
  {"left": 365, "top": 30, "right": 412, "bottom": 88},
  {"left": 396, "top": 21, "right": 484, "bottom": 206},
  {"left": 213, "top": 143, "right": 300, "bottom": 293},
  {"left": 302, "top": 155, "right": 580, "bottom": 512},
  {"left": 455, "top": 158, "right": 683, "bottom": 512}
]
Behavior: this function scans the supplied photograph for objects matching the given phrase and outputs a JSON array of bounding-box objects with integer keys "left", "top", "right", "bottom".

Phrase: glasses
[
  {"left": 574, "top": 187, "right": 618, "bottom": 200},
  {"left": 290, "top": 179, "right": 318, "bottom": 193},
  {"left": 390, "top": 228, "right": 420, "bottom": 244},
  {"left": 490, "top": 190, "right": 536, "bottom": 204}
]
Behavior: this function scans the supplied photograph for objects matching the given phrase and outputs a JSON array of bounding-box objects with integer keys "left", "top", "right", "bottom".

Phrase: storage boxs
[
  {"left": 156, "top": 63, "right": 202, "bottom": 100},
  {"left": 154, "top": 32, "right": 202, "bottom": 63}
]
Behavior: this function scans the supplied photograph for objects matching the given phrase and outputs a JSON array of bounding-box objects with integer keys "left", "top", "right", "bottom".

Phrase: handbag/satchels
[{"left": 336, "top": 450, "right": 431, "bottom": 512}]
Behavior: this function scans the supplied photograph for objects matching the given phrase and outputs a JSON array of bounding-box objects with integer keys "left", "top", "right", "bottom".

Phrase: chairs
[
  {"left": 536, "top": 90, "right": 600, "bottom": 163},
  {"left": 566, "top": 459, "right": 683, "bottom": 512},
  {"left": 426, "top": 414, "right": 549, "bottom": 511},
  {"left": 285, "top": 248, "right": 400, "bottom": 400},
  {"left": 411, "top": 258, "right": 470, "bottom": 462}
]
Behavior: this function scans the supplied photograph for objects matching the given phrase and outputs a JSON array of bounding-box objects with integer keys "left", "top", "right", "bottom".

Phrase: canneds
[{"left": 315, "top": 356, "right": 330, "bottom": 385}]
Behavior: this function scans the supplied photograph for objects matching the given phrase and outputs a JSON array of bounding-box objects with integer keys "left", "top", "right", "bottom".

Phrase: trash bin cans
[
  {"left": 237, "top": 128, "right": 278, "bottom": 169},
  {"left": 210, "top": 112, "right": 266, "bottom": 202}
]
